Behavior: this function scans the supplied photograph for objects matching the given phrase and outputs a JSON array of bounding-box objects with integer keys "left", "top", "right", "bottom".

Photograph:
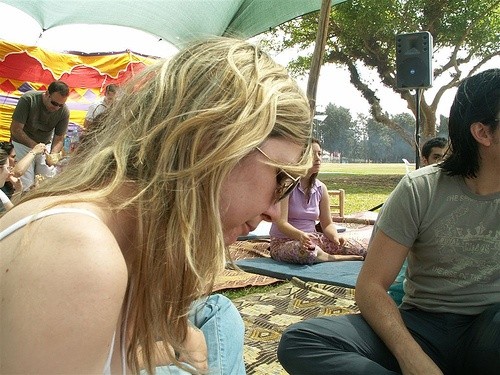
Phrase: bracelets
[{"left": 29, "top": 151, "right": 35, "bottom": 156}]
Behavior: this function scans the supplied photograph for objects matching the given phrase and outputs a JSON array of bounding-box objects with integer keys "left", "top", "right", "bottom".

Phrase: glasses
[
  {"left": 255, "top": 147, "right": 302, "bottom": 202},
  {"left": 49, "top": 96, "right": 64, "bottom": 108}
]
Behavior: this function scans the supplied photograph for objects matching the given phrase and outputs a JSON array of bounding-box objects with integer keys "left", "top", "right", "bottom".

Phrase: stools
[{"left": 327, "top": 189, "right": 345, "bottom": 217}]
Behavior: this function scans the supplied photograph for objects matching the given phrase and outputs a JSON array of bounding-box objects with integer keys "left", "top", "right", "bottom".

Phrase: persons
[
  {"left": 421, "top": 136, "right": 449, "bottom": 166},
  {"left": 275, "top": 68, "right": 500, "bottom": 375},
  {"left": 0, "top": 37, "right": 312, "bottom": 375},
  {"left": 269, "top": 139, "right": 371, "bottom": 265}
]
